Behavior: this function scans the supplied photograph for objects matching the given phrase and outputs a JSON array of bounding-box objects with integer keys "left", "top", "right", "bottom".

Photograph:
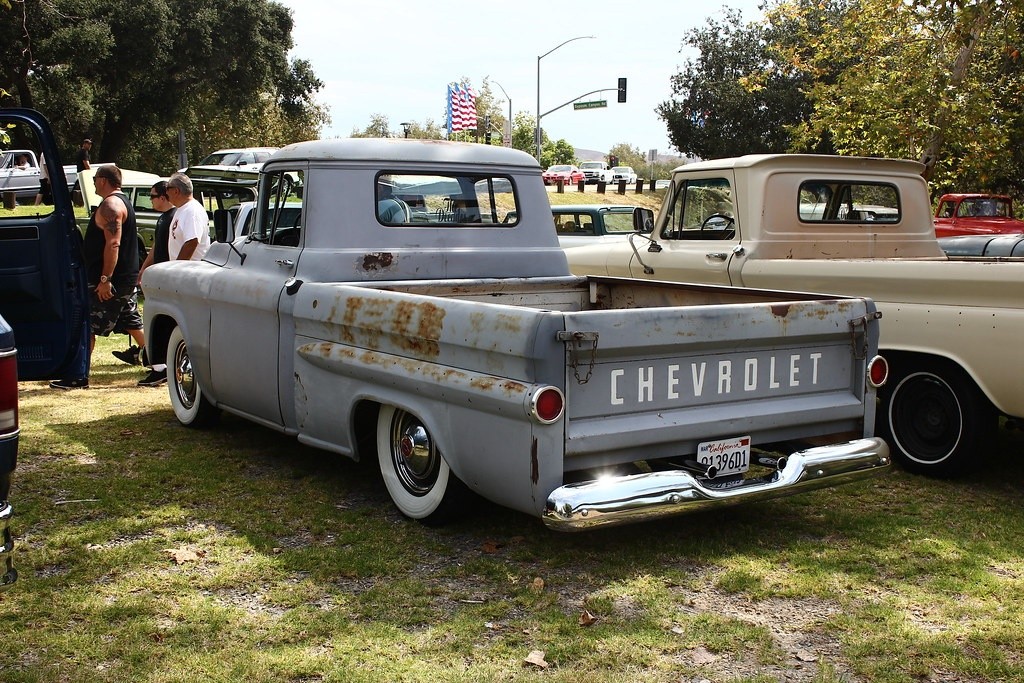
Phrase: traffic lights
[
  {"left": 486, "top": 133, "right": 491, "bottom": 144},
  {"left": 610, "top": 155, "right": 619, "bottom": 167},
  {"left": 485, "top": 115, "right": 491, "bottom": 129}
]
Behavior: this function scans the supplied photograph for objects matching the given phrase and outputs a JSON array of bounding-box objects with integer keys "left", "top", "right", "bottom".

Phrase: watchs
[{"left": 100, "top": 275, "right": 111, "bottom": 283}]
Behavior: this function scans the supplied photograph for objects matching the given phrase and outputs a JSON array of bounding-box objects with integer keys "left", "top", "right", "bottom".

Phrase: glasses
[
  {"left": 93, "top": 176, "right": 109, "bottom": 182},
  {"left": 149, "top": 194, "right": 165, "bottom": 200},
  {"left": 165, "top": 185, "right": 180, "bottom": 192}
]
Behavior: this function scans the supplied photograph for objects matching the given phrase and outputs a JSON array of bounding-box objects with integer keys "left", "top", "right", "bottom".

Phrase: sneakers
[
  {"left": 50, "top": 376, "right": 88, "bottom": 390},
  {"left": 138, "top": 367, "right": 168, "bottom": 386},
  {"left": 112, "top": 345, "right": 140, "bottom": 365}
]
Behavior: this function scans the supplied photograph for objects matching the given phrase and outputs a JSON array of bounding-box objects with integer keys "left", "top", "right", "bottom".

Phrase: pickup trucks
[
  {"left": 0, "top": 150, "right": 115, "bottom": 198},
  {"left": 557, "top": 153, "right": 1024, "bottom": 474},
  {"left": 75, "top": 147, "right": 305, "bottom": 271},
  {"left": 392, "top": 194, "right": 1024, "bottom": 257},
  {"left": 140, "top": 137, "right": 891, "bottom": 533}
]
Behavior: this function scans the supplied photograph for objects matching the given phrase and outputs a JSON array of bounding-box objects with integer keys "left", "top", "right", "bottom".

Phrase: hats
[{"left": 84, "top": 139, "right": 92, "bottom": 144}]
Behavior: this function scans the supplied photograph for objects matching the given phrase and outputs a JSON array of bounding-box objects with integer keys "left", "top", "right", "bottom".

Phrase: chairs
[
  {"left": 561, "top": 221, "right": 576, "bottom": 232},
  {"left": 583, "top": 222, "right": 593, "bottom": 230}
]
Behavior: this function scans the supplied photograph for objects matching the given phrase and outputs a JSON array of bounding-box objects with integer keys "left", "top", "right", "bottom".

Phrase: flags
[{"left": 448, "top": 83, "right": 478, "bottom": 132}]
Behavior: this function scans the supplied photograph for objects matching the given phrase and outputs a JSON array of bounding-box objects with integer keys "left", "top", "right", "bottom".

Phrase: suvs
[
  {"left": 580, "top": 161, "right": 615, "bottom": 184},
  {"left": 0, "top": 108, "right": 91, "bottom": 586}
]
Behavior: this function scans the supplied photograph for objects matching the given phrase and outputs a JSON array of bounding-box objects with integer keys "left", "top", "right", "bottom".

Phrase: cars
[
  {"left": 610, "top": 166, "right": 637, "bottom": 184},
  {"left": 543, "top": 164, "right": 586, "bottom": 185}
]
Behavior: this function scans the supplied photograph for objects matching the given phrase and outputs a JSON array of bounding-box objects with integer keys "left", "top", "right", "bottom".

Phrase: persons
[
  {"left": 50, "top": 165, "right": 144, "bottom": 388},
  {"left": 138, "top": 173, "right": 210, "bottom": 385},
  {"left": 35, "top": 152, "right": 54, "bottom": 205},
  {"left": 14, "top": 154, "right": 31, "bottom": 169},
  {"left": 112, "top": 181, "right": 178, "bottom": 365},
  {"left": 70, "top": 139, "right": 92, "bottom": 207},
  {"left": 379, "top": 176, "right": 413, "bottom": 223}
]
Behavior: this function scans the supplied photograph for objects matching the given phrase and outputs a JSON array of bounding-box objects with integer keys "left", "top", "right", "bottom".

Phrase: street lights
[
  {"left": 491, "top": 80, "right": 511, "bottom": 147},
  {"left": 400, "top": 123, "right": 411, "bottom": 138},
  {"left": 536, "top": 35, "right": 598, "bottom": 164}
]
